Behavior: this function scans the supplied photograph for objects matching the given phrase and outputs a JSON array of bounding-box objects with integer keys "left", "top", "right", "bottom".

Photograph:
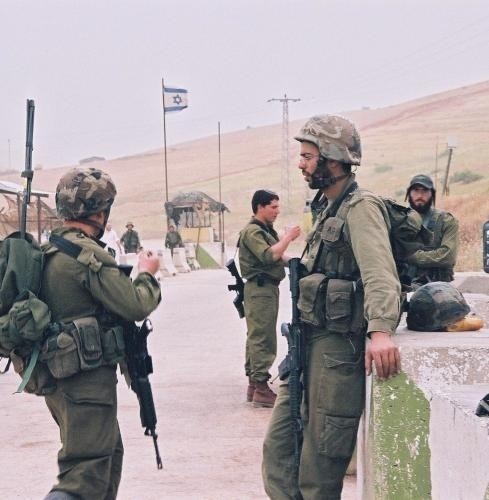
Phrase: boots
[
  {"left": 246, "top": 377, "right": 255, "bottom": 403},
  {"left": 252, "top": 380, "right": 277, "bottom": 408}
]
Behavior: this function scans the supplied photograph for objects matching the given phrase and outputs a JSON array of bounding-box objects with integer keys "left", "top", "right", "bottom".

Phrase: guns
[
  {"left": 21, "top": 99, "right": 35, "bottom": 240},
  {"left": 277, "top": 257, "right": 303, "bottom": 462},
  {"left": 226, "top": 259, "right": 245, "bottom": 318},
  {"left": 118, "top": 265, "right": 162, "bottom": 470}
]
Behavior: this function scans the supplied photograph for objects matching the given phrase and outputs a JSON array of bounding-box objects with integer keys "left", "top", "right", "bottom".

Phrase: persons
[
  {"left": 38, "top": 166, "right": 161, "bottom": 500},
  {"left": 120, "top": 220, "right": 143, "bottom": 256},
  {"left": 237, "top": 188, "right": 302, "bottom": 409},
  {"left": 260, "top": 113, "right": 404, "bottom": 500},
  {"left": 101, "top": 223, "right": 123, "bottom": 258},
  {"left": 165, "top": 225, "right": 184, "bottom": 257},
  {"left": 404, "top": 170, "right": 459, "bottom": 289}
]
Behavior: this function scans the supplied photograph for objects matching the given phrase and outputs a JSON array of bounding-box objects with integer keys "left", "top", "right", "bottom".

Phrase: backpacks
[
  {"left": 0, "top": 230, "right": 43, "bottom": 358},
  {"left": 4, "top": 294, "right": 58, "bottom": 394}
]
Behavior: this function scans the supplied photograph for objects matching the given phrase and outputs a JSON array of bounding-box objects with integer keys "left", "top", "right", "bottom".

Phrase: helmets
[
  {"left": 294, "top": 113, "right": 361, "bottom": 165},
  {"left": 406, "top": 281, "right": 470, "bottom": 331},
  {"left": 55, "top": 166, "right": 117, "bottom": 218}
]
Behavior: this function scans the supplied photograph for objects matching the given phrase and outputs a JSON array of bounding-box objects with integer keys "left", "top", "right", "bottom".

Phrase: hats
[
  {"left": 125, "top": 221, "right": 134, "bottom": 228},
  {"left": 406, "top": 175, "right": 433, "bottom": 190}
]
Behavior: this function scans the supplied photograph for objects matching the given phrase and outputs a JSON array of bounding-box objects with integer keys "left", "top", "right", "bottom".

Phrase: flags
[{"left": 163, "top": 87, "right": 189, "bottom": 112}]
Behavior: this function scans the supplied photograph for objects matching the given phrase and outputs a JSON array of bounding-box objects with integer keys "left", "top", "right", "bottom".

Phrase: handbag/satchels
[
  {"left": 297, "top": 274, "right": 364, "bottom": 337},
  {"left": 45, "top": 316, "right": 127, "bottom": 377}
]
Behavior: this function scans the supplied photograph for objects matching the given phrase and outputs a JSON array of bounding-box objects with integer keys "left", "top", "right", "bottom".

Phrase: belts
[{"left": 248, "top": 276, "right": 280, "bottom": 286}]
